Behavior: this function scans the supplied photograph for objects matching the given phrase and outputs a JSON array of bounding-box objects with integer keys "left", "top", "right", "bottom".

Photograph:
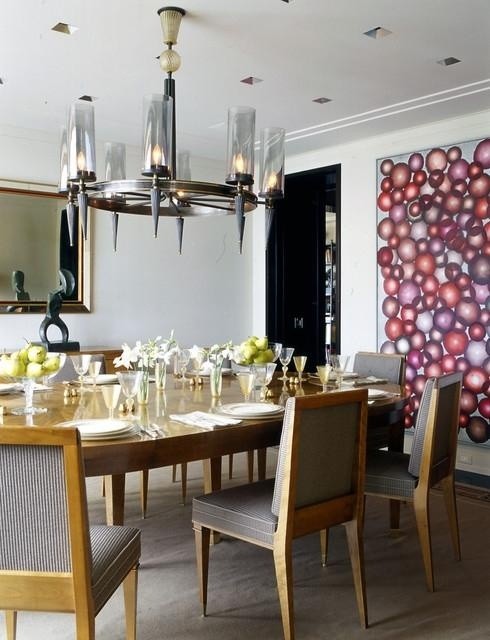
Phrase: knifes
[{"left": 136, "top": 422, "right": 167, "bottom": 439}]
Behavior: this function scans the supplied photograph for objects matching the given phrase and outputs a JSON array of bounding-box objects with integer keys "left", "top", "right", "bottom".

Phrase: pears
[
  {"left": 237, "top": 334, "right": 274, "bottom": 366},
  {"left": 0, "top": 346, "right": 60, "bottom": 379}
]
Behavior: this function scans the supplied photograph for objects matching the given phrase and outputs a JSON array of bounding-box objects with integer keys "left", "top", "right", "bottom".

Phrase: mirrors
[{"left": 0, "top": 179, "right": 93, "bottom": 315}]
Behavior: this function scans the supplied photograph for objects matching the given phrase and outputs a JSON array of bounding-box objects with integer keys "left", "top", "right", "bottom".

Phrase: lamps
[{"left": 59, "top": 5, "right": 285, "bottom": 254}]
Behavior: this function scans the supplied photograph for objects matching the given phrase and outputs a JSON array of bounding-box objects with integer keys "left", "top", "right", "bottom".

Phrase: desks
[{"left": 6, "top": 364, "right": 416, "bottom": 587}]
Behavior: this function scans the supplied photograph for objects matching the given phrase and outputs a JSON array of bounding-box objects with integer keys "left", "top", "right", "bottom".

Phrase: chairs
[
  {"left": 342, "top": 351, "right": 410, "bottom": 532},
  {"left": 71, "top": 350, "right": 152, "bottom": 526},
  {"left": 187, "top": 386, "right": 376, "bottom": 638},
  {"left": 311, "top": 370, "right": 462, "bottom": 595},
  {"left": 172, "top": 449, "right": 256, "bottom": 500},
  {"left": 0, "top": 421, "right": 144, "bottom": 640}
]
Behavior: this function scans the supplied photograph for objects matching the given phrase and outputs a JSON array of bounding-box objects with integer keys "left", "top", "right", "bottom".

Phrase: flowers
[{"left": 114, "top": 330, "right": 241, "bottom": 370}]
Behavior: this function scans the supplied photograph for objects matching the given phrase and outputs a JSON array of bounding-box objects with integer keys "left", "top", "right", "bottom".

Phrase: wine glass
[
  {"left": 101, "top": 384, "right": 121, "bottom": 419},
  {"left": 277, "top": 348, "right": 294, "bottom": 380},
  {"left": 116, "top": 371, "right": 144, "bottom": 420},
  {"left": 295, "top": 380, "right": 305, "bottom": 396},
  {"left": 330, "top": 355, "right": 351, "bottom": 390},
  {"left": 70, "top": 354, "right": 91, "bottom": 391},
  {"left": 316, "top": 366, "right": 332, "bottom": 394},
  {"left": 292, "top": 356, "right": 308, "bottom": 381},
  {"left": 237, "top": 373, "right": 258, "bottom": 402},
  {"left": 278, "top": 380, "right": 291, "bottom": 406},
  {"left": 88, "top": 362, "right": 102, "bottom": 390},
  {"left": 0, "top": 352, "right": 67, "bottom": 415},
  {"left": 176, "top": 351, "right": 190, "bottom": 382}
]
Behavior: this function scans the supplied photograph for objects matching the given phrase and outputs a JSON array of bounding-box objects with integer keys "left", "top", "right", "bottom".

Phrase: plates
[
  {"left": 53, "top": 419, "right": 139, "bottom": 440},
  {"left": 354, "top": 388, "right": 387, "bottom": 399},
  {"left": 78, "top": 374, "right": 119, "bottom": 384},
  {"left": 0, "top": 383, "right": 16, "bottom": 394},
  {"left": 220, "top": 402, "right": 284, "bottom": 416}
]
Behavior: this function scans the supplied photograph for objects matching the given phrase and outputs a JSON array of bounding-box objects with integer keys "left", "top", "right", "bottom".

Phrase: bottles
[
  {"left": 210, "top": 367, "right": 223, "bottom": 397},
  {"left": 155, "top": 362, "right": 167, "bottom": 390},
  {"left": 137, "top": 372, "right": 150, "bottom": 405}
]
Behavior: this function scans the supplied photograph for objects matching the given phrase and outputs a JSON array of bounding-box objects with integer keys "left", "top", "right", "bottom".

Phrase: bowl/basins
[
  {"left": 227, "top": 342, "right": 282, "bottom": 376},
  {"left": 234, "top": 362, "right": 277, "bottom": 390}
]
